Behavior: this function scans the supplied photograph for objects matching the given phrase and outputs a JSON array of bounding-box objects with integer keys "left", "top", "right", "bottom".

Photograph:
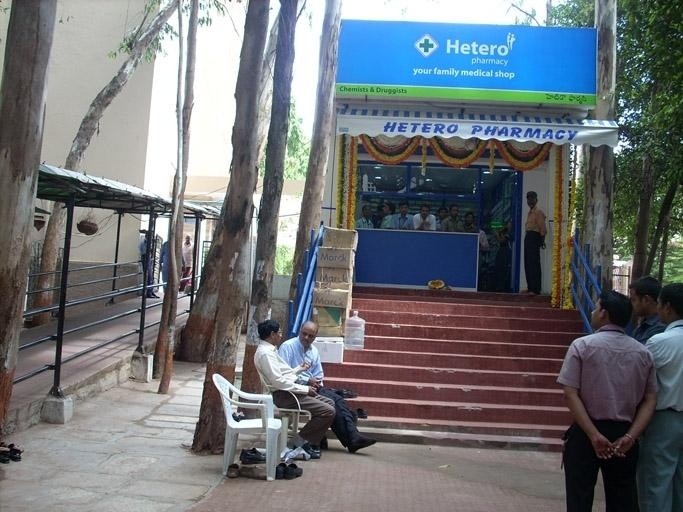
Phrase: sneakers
[
  {"left": 357, "top": 408, "right": 367, "bottom": 418},
  {"left": 240, "top": 466, "right": 267, "bottom": 480},
  {"left": 9, "top": 442, "right": 22, "bottom": 461},
  {"left": 0, "top": 443, "right": 10, "bottom": 463},
  {"left": 227, "top": 463, "right": 240, "bottom": 478}
]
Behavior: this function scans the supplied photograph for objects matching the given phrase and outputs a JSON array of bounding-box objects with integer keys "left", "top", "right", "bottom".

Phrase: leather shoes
[
  {"left": 347, "top": 433, "right": 377, "bottom": 453},
  {"left": 276, "top": 463, "right": 287, "bottom": 479},
  {"left": 293, "top": 442, "right": 321, "bottom": 458},
  {"left": 147, "top": 294, "right": 159, "bottom": 298},
  {"left": 242, "top": 450, "right": 266, "bottom": 464},
  {"left": 340, "top": 390, "right": 357, "bottom": 398},
  {"left": 240, "top": 448, "right": 266, "bottom": 460},
  {"left": 285, "top": 463, "right": 303, "bottom": 479}
]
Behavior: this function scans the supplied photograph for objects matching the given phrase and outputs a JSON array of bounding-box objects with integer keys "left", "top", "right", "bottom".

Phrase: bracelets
[{"left": 624, "top": 433, "right": 635, "bottom": 444}]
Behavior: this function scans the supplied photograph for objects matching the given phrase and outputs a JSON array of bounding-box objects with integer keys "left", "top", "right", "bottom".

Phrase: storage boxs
[{"left": 312, "top": 226, "right": 358, "bottom": 362}]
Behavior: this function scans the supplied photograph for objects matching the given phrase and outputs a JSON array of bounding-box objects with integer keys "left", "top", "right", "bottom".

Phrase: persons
[
  {"left": 436, "top": 208, "right": 448, "bottom": 230},
  {"left": 137, "top": 232, "right": 157, "bottom": 298},
  {"left": 463, "top": 211, "right": 478, "bottom": 233},
  {"left": 379, "top": 200, "right": 392, "bottom": 229},
  {"left": 441, "top": 205, "right": 464, "bottom": 233},
  {"left": 252, "top": 319, "right": 336, "bottom": 460},
  {"left": 414, "top": 205, "right": 435, "bottom": 231},
  {"left": 628, "top": 284, "right": 644, "bottom": 328},
  {"left": 519, "top": 191, "right": 546, "bottom": 296},
  {"left": 177, "top": 235, "right": 192, "bottom": 290},
  {"left": 278, "top": 320, "right": 377, "bottom": 453},
  {"left": 637, "top": 282, "right": 683, "bottom": 511},
  {"left": 629, "top": 278, "right": 664, "bottom": 344},
  {"left": 355, "top": 205, "right": 374, "bottom": 228},
  {"left": 158, "top": 239, "right": 187, "bottom": 295},
  {"left": 553, "top": 290, "right": 659, "bottom": 511},
  {"left": 389, "top": 200, "right": 413, "bottom": 230}
]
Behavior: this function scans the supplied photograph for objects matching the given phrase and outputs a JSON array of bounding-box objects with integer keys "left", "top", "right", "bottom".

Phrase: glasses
[{"left": 300, "top": 331, "right": 315, "bottom": 338}]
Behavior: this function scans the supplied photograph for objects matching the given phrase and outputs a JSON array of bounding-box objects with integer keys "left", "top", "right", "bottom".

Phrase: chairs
[
  {"left": 211, "top": 373, "right": 286, "bottom": 482},
  {"left": 254, "top": 366, "right": 316, "bottom": 452}
]
